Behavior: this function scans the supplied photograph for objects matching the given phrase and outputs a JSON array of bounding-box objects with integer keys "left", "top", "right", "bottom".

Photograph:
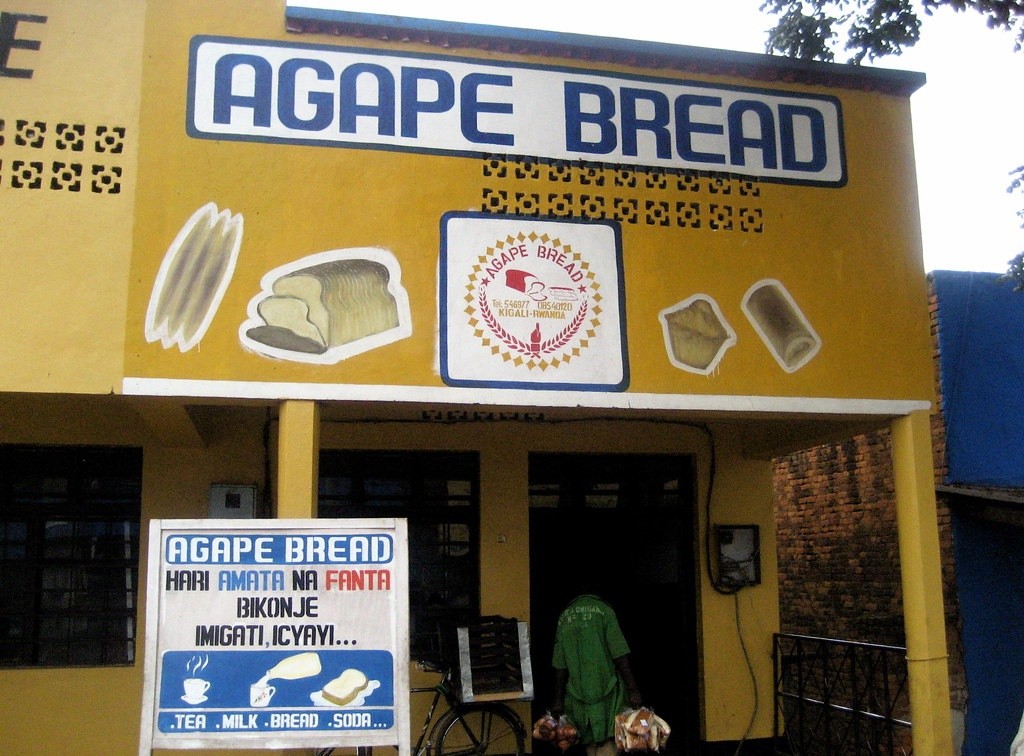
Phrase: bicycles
[{"left": 314, "top": 658, "right": 529, "bottom": 756}]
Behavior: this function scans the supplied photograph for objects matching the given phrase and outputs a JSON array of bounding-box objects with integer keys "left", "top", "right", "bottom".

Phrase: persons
[{"left": 552, "top": 594, "right": 641, "bottom": 756}]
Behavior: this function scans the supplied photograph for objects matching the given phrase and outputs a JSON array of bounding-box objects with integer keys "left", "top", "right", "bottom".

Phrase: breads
[
  {"left": 615, "top": 708, "right": 671, "bottom": 752},
  {"left": 533, "top": 718, "right": 578, "bottom": 751}
]
